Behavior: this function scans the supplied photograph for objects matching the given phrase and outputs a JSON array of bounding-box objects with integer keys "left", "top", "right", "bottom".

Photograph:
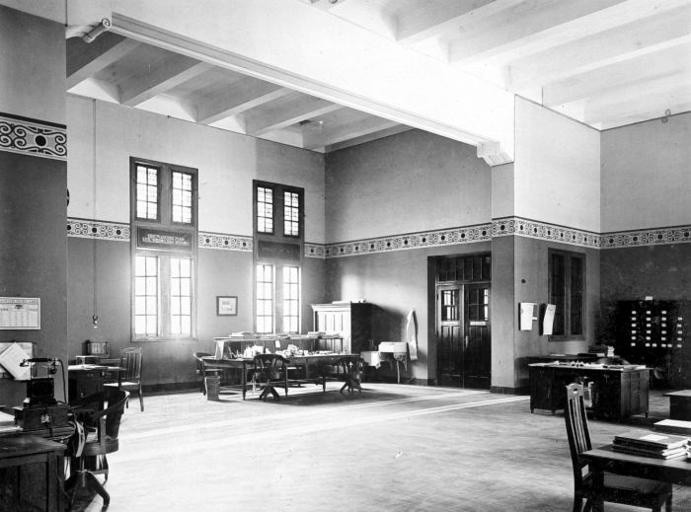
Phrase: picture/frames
[{"left": 216, "top": 296, "right": 238, "bottom": 316}]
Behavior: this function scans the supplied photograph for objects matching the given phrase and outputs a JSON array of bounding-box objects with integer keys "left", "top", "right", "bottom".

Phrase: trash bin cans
[{"left": 204, "top": 376, "right": 220, "bottom": 401}]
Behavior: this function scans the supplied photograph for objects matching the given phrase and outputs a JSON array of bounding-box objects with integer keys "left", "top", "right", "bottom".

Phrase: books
[{"left": 612, "top": 428, "right": 691, "bottom": 462}]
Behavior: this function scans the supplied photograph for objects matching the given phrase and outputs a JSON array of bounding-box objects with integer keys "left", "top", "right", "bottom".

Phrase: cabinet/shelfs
[
  {"left": 611, "top": 294, "right": 690, "bottom": 387},
  {"left": 312, "top": 302, "right": 369, "bottom": 379}
]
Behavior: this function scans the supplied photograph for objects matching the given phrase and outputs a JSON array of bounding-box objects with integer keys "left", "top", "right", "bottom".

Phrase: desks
[
  {"left": 68, "top": 365, "right": 129, "bottom": 408},
  {"left": 521, "top": 352, "right": 654, "bottom": 421},
  {"left": 581, "top": 390, "right": 691, "bottom": 512},
  {"left": 0, "top": 416, "right": 74, "bottom": 511}
]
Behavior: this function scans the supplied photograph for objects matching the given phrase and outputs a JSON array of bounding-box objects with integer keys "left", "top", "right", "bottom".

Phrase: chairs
[
  {"left": 191, "top": 349, "right": 361, "bottom": 400},
  {"left": 561, "top": 382, "right": 671, "bottom": 511},
  {"left": 101, "top": 347, "right": 145, "bottom": 411},
  {"left": 59, "top": 388, "right": 130, "bottom": 512}
]
